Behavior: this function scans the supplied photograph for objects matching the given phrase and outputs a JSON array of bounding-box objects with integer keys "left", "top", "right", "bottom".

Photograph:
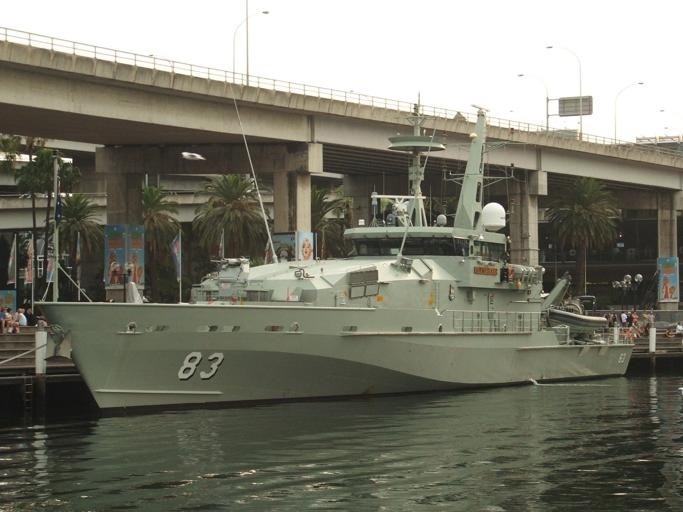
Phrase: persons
[
  {"left": 647, "top": 311, "right": 655, "bottom": 324},
  {"left": 301, "top": 239, "right": 312, "bottom": 260},
  {"left": 603, "top": 309, "right": 639, "bottom": 328},
  {"left": 676, "top": 321, "right": 683, "bottom": 336},
  {"left": 1, "top": 306, "right": 47, "bottom": 335}
]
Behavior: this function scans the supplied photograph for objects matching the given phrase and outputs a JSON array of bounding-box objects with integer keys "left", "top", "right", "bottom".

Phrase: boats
[{"left": 33, "top": 90, "right": 635, "bottom": 411}]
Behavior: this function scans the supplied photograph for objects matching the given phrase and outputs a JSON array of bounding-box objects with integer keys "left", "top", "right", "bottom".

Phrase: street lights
[
  {"left": 545, "top": 44, "right": 583, "bottom": 137},
  {"left": 232, "top": 9, "right": 269, "bottom": 86}
]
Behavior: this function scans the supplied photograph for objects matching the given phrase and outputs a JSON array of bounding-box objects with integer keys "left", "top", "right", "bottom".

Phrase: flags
[
  {"left": 263, "top": 237, "right": 278, "bottom": 264},
  {"left": 74, "top": 232, "right": 82, "bottom": 281},
  {"left": 218, "top": 230, "right": 225, "bottom": 258},
  {"left": 44, "top": 232, "right": 57, "bottom": 284},
  {"left": 5, "top": 234, "right": 17, "bottom": 286},
  {"left": 23, "top": 234, "right": 34, "bottom": 284},
  {"left": 167, "top": 229, "right": 183, "bottom": 283}
]
